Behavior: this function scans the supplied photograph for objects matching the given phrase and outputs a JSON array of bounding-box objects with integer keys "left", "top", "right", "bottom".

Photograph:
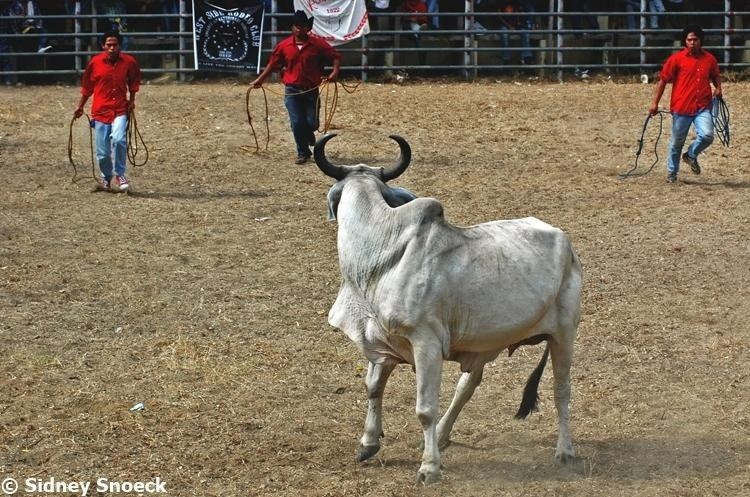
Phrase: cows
[{"left": 313, "top": 133, "right": 582, "bottom": 485}]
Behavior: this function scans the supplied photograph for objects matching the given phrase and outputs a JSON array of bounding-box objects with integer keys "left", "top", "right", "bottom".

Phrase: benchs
[{"left": 0, "top": 0, "right": 750, "bottom": 74}]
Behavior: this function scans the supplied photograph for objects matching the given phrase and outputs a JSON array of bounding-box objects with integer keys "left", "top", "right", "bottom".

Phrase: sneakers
[
  {"left": 296, "top": 155, "right": 310, "bottom": 164},
  {"left": 666, "top": 176, "right": 676, "bottom": 183},
  {"left": 309, "top": 133, "right": 315, "bottom": 147},
  {"left": 682, "top": 153, "right": 700, "bottom": 174},
  {"left": 103, "top": 176, "right": 112, "bottom": 189},
  {"left": 114, "top": 176, "right": 129, "bottom": 191}
]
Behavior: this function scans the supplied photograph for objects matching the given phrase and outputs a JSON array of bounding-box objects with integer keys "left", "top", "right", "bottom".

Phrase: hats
[{"left": 285, "top": 10, "right": 314, "bottom": 30}]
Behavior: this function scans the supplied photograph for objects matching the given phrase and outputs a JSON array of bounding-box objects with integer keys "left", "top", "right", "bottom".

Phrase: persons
[
  {"left": 72, "top": 30, "right": 143, "bottom": 193},
  {"left": 647, "top": 21, "right": 722, "bottom": 184},
  {"left": 367, "top": 1, "right": 750, "bottom": 71},
  {"left": 1, "top": 0, "right": 182, "bottom": 88},
  {"left": 247, "top": 8, "right": 343, "bottom": 164}
]
